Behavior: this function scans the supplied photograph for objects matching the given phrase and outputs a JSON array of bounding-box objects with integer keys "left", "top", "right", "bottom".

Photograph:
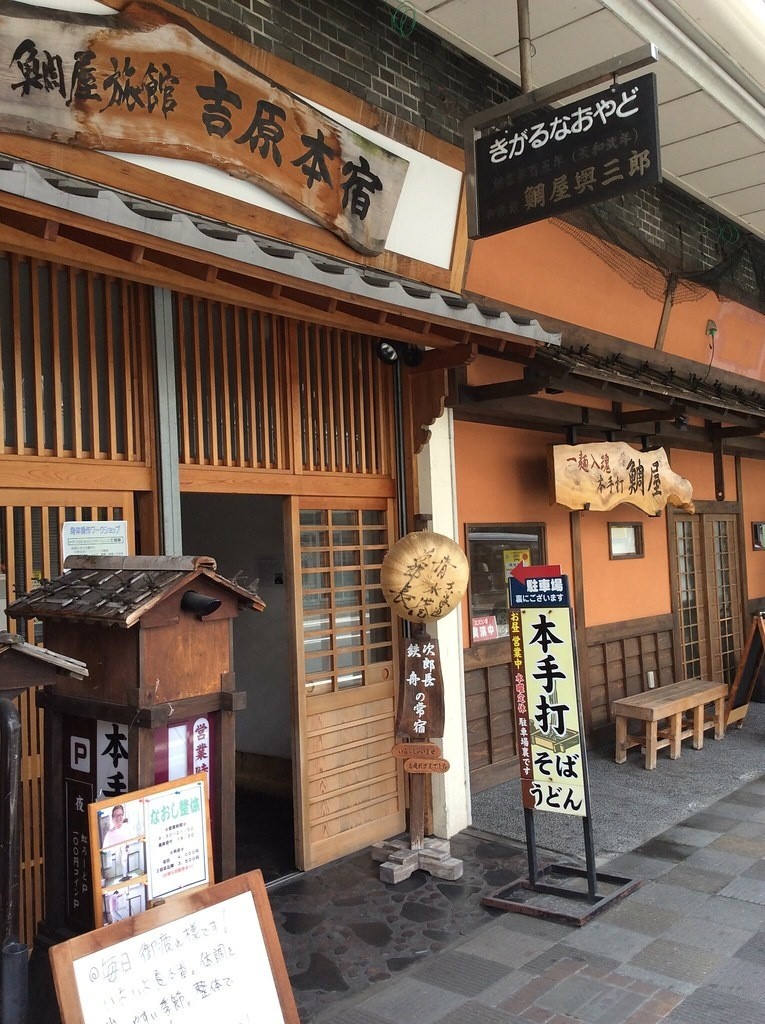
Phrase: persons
[{"left": 102, "top": 803, "right": 140, "bottom": 923}]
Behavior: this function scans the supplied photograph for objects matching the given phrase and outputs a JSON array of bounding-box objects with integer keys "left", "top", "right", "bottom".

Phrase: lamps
[
  {"left": 673, "top": 416, "right": 689, "bottom": 432},
  {"left": 377, "top": 337, "right": 422, "bottom": 367}
]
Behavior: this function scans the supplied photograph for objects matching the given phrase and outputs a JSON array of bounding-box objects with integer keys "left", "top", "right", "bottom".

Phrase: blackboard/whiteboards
[
  {"left": 48, "top": 867, "right": 299, "bottom": 1024},
  {"left": 724, "top": 610, "right": 765, "bottom": 724}
]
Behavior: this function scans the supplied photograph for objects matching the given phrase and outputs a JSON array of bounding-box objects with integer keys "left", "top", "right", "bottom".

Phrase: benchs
[{"left": 611, "top": 677, "right": 729, "bottom": 771}]
